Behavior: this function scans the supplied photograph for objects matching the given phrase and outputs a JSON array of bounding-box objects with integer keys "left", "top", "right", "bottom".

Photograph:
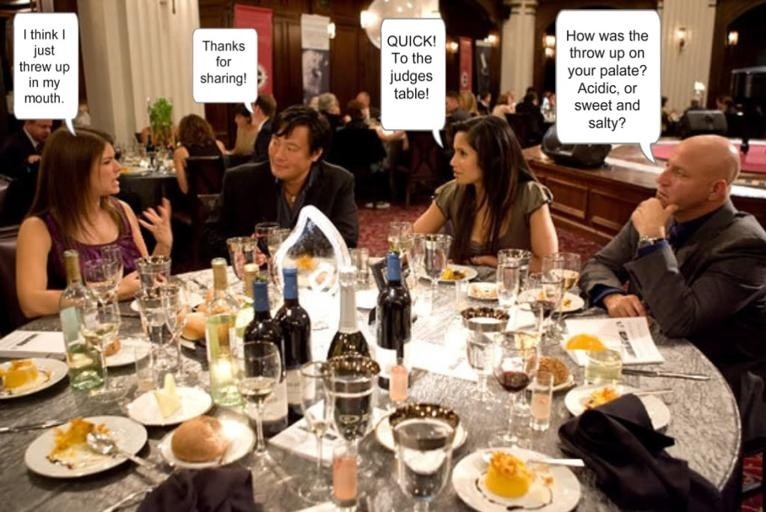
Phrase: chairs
[
  {"left": 1, "top": 225, "right": 22, "bottom": 314},
  {"left": 394, "top": 129, "right": 439, "bottom": 208},
  {"left": 175, "top": 155, "right": 226, "bottom": 245}
]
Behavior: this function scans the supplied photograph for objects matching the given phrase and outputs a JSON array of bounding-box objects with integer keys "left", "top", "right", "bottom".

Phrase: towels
[
  {"left": 135, "top": 464, "right": 257, "bottom": 512},
  {"left": 557, "top": 392, "right": 725, "bottom": 512}
]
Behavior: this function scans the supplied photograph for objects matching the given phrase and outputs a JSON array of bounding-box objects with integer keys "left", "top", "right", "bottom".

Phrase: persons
[
  {"left": 663, "top": 95, "right": 765, "bottom": 130},
  {"left": 317, "top": 92, "right": 339, "bottom": 125},
  {"left": 401, "top": 115, "right": 558, "bottom": 275},
  {"left": 516, "top": 93, "right": 545, "bottom": 142},
  {"left": 495, "top": 93, "right": 512, "bottom": 115},
  {"left": 356, "top": 92, "right": 380, "bottom": 119},
  {"left": 173, "top": 114, "right": 228, "bottom": 194},
  {"left": 336, "top": 101, "right": 386, "bottom": 159},
  {"left": 7, "top": 119, "right": 53, "bottom": 177},
  {"left": 445, "top": 90, "right": 492, "bottom": 122},
  {"left": 251, "top": 95, "right": 277, "bottom": 164},
  {"left": 578, "top": 134, "right": 765, "bottom": 339},
  {"left": 16, "top": 127, "right": 173, "bottom": 318},
  {"left": 218, "top": 105, "right": 359, "bottom": 250},
  {"left": 217, "top": 103, "right": 259, "bottom": 156}
]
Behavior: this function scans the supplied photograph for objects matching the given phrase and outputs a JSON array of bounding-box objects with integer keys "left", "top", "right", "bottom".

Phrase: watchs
[{"left": 636, "top": 234, "right": 670, "bottom": 248}]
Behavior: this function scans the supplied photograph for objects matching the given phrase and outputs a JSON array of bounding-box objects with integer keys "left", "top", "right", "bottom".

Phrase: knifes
[
  {"left": 621, "top": 369, "right": 711, "bottom": 381},
  {"left": 0, "top": 417, "right": 66, "bottom": 435}
]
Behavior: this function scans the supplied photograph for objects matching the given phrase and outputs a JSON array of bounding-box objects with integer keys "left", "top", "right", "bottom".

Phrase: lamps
[
  {"left": 676, "top": 26, "right": 687, "bottom": 48},
  {"left": 726, "top": 29, "right": 739, "bottom": 48}
]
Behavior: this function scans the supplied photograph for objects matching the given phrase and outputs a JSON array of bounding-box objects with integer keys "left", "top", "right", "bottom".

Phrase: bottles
[
  {"left": 146, "top": 134, "right": 156, "bottom": 168},
  {"left": 327, "top": 268, "right": 373, "bottom": 443},
  {"left": 274, "top": 267, "right": 316, "bottom": 418},
  {"left": 59, "top": 250, "right": 105, "bottom": 389},
  {"left": 243, "top": 279, "right": 288, "bottom": 436},
  {"left": 375, "top": 254, "right": 412, "bottom": 390},
  {"left": 204, "top": 258, "right": 247, "bottom": 406}
]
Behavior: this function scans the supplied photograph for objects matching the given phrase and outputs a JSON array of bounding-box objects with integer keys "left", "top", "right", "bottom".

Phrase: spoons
[
  {"left": 482, "top": 451, "right": 585, "bottom": 468},
  {"left": 86, "top": 430, "right": 157, "bottom": 471}
]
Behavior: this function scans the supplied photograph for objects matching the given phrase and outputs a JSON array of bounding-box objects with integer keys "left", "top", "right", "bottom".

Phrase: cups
[
  {"left": 529, "top": 371, "right": 555, "bottom": 431},
  {"left": 244, "top": 265, "right": 260, "bottom": 299},
  {"left": 426, "top": 234, "right": 452, "bottom": 307},
  {"left": 542, "top": 251, "right": 581, "bottom": 320},
  {"left": 387, "top": 222, "right": 413, "bottom": 253},
  {"left": 74, "top": 295, "right": 127, "bottom": 404},
  {"left": 226, "top": 238, "right": 258, "bottom": 281},
  {"left": 493, "top": 332, "right": 539, "bottom": 448},
  {"left": 333, "top": 445, "right": 359, "bottom": 508},
  {"left": 413, "top": 232, "right": 425, "bottom": 273},
  {"left": 455, "top": 279, "right": 469, "bottom": 316},
  {"left": 390, "top": 404, "right": 460, "bottom": 512},
  {"left": 584, "top": 348, "right": 622, "bottom": 383},
  {"left": 497, "top": 248, "right": 532, "bottom": 295},
  {"left": 255, "top": 224, "right": 282, "bottom": 257},
  {"left": 84, "top": 259, "right": 122, "bottom": 288},
  {"left": 352, "top": 248, "right": 369, "bottom": 288},
  {"left": 134, "top": 286, "right": 161, "bottom": 336},
  {"left": 389, "top": 358, "right": 409, "bottom": 407},
  {"left": 495, "top": 263, "right": 520, "bottom": 312},
  {"left": 529, "top": 272, "right": 565, "bottom": 346},
  {"left": 134, "top": 256, "right": 171, "bottom": 288},
  {"left": 326, "top": 355, "right": 381, "bottom": 475},
  {"left": 160, "top": 285, "right": 191, "bottom": 383},
  {"left": 212, "top": 258, "right": 227, "bottom": 291},
  {"left": 268, "top": 227, "right": 291, "bottom": 257},
  {"left": 461, "top": 308, "right": 510, "bottom": 402},
  {"left": 300, "top": 361, "right": 335, "bottom": 493},
  {"left": 102, "top": 246, "right": 124, "bottom": 319},
  {"left": 230, "top": 342, "right": 281, "bottom": 472}
]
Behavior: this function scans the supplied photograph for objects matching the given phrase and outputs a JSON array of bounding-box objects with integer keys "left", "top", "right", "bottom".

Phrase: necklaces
[{"left": 283, "top": 186, "right": 296, "bottom": 203}]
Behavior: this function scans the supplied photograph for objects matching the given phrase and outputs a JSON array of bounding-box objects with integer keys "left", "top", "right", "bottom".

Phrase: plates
[
  {"left": 24, "top": 414, "right": 148, "bottom": 479},
  {"left": 131, "top": 291, "right": 203, "bottom": 313},
  {"left": 104, "top": 338, "right": 151, "bottom": 369},
  {"left": 355, "top": 287, "right": 415, "bottom": 309},
  {"left": 128, "top": 386, "right": 214, "bottom": 425},
  {"left": 374, "top": 414, "right": 467, "bottom": 454},
  {"left": 468, "top": 282, "right": 498, "bottom": 300},
  {"left": 525, "top": 374, "right": 574, "bottom": 391},
  {"left": 159, "top": 417, "right": 255, "bottom": 468},
  {"left": 518, "top": 289, "right": 586, "bottom": 313},
  {"left": 421, "top": 264, "right": 479, "bottom": 282},
  {"left": 452, "top": 446, "right": 581, "bottom": 512},
  {"left": 0, "top": 357, "right": 69, "bottom": 399},
  {"left": 564, "top": 384, "right": 670, "bottom": 431}
]
